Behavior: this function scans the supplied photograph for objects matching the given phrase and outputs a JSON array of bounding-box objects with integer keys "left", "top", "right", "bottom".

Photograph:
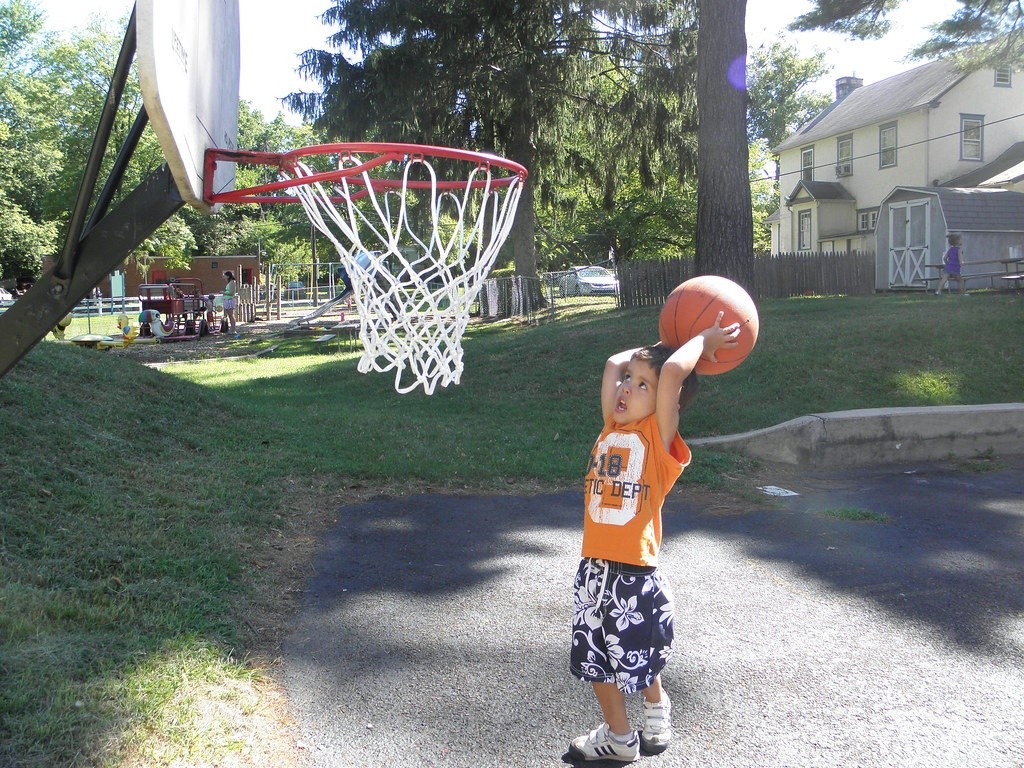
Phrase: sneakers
[
  {"left": 570, "top": 723, "right": 640, "bottom": 763},
  {"left": 642, "top": 687, "right": 671, "bottom": 754}
]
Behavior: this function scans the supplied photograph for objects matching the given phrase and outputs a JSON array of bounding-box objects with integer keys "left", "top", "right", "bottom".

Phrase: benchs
[
  {"left": 173, "top": 277, "right": 209, "bottom": 337},
  {"left": 915, "top": 258, "right": 1024, "bottom": 293},
  {"left": 137, "top": 284, "right": 185, "bottom": 337},
  {"left": 314, "top": 334, "right": 337, "bottom": 354}
]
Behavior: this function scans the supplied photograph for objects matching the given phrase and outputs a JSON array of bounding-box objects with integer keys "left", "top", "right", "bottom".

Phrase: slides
[{"left": 288, "top": 286, "right": 355, "bottom": 329}]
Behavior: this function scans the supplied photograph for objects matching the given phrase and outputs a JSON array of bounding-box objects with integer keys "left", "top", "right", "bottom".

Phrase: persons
[
  {"left": 193, "top": 289, "right": 216, "bottom": 330},
  {"left": 219, "top": 271, "right": 238, "bottom": 333},
  {"left": 569, "top": 310, "right": 739, "bottom": 760},
  {"left": 935, "top": 234, "right": 965, "bottom": 295},
  {"left": 176, "top": 289, "right": 188, "bottom": 326}
]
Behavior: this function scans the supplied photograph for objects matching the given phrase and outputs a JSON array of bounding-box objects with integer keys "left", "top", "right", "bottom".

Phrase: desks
[{"left": 331, "top": 323, "right": 361, "bottom": 352}]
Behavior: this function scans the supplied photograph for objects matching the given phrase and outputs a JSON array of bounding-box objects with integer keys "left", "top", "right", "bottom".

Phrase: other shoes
[
  {"left": 935, "top": 291, "right": 941, "bottom": 295},
  {"left": 961, "top": 293, "right": 969, "bottom": 296}
]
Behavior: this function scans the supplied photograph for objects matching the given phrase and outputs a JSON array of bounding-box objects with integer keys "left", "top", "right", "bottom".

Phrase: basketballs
[{"left": 657, "top": 273, "right": 762, "bottom": 378}]
